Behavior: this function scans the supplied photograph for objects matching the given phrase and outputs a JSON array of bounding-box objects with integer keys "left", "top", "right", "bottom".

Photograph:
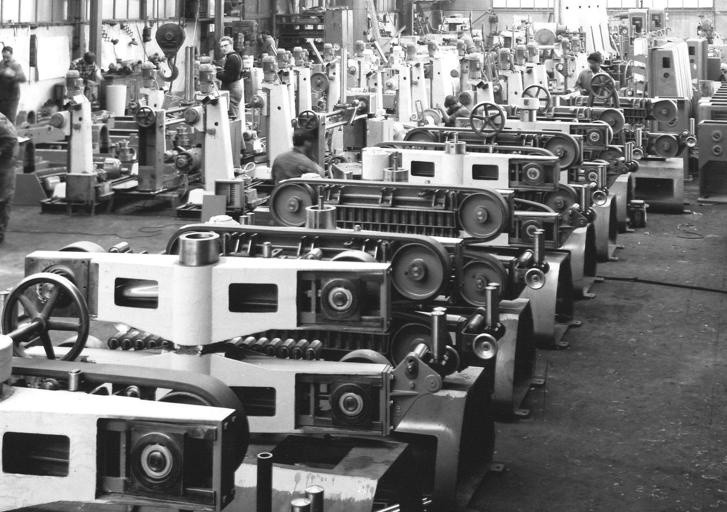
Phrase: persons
[
  {"left": 214, "top": 36, "right": 243, "bottom": 120},
  {"left": 441, "top": 95, "right": 463, "bottom": 127},
  {"left": 572, "top": 52, "right": 608, "bottom": 96},
  {"left": 268, "top": 128, "right": 325, "bottom": 184},
  {"left": 0, "top": 111, "right": 20, "bottom": 243},
  {"left": 69, "top": 50, "right": 104, "bottom": 85},
  {"left": 0, "top": 46, "right": 27, "bottom": 124},
  {"left": 434, "top": 92, "right": 473, "bottom": 124},
  {"left": 260, "top": 30, "right": 276, "bottom": 57}
]
